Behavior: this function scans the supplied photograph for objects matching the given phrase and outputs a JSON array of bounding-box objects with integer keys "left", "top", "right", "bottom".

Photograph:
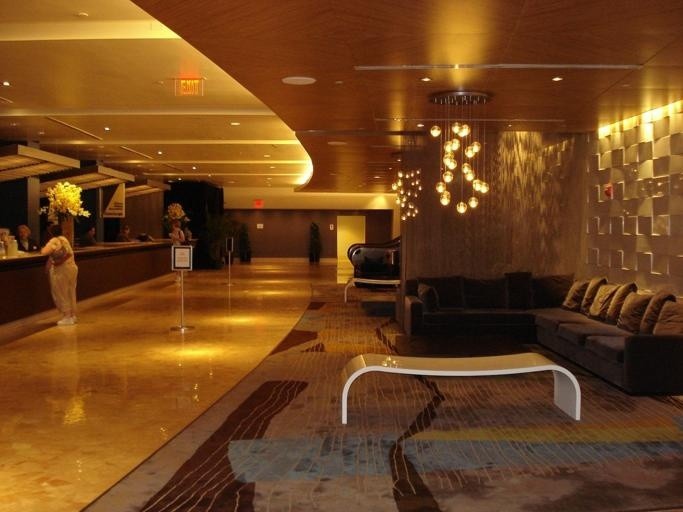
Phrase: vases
[{"left": 58, "top": 215, "right": 74, "bottom": 249}]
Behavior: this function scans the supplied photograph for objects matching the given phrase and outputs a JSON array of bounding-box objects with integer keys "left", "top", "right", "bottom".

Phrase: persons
[
  {"left": 309, "top": 220, "right": 321, "bottom": 263},
  {"left": 116, "top": 225, "right": 131, "bottom": 242},
  {"left": 40, "top": 223, "right": 78, "bottom": 326},
  {"left": 46, "top": 324, "right": 81, "bottom": 448},
  {"left": 80, "top": 226, "right": 98, "bottom": 247},
  {"left": 41, "top": 221, "right": 55, "bottom": 247},
  {"left": 169, "top": 221, "right": 185, "bottom": 283},
  {"left": 237, "top": 222, "right": 252, "bottom": 264},
  {"left": 183, "top": 217, "right": 194, "bottom": 246},
  {"left": 16, "top": 225, "right": 38, "bottom": 252}
]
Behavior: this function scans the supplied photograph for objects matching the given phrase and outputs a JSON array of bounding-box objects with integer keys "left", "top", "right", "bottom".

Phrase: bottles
[{"left": 0, "top": 240, "right": 18, "bottom": 259}]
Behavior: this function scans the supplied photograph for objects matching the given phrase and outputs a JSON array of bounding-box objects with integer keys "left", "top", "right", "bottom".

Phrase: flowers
[
  {"left": 44, "top": 180, "right": 92, "bottom": 227},
  {"left": 167, "top": 203, "right": 187, "bottom": 220}
]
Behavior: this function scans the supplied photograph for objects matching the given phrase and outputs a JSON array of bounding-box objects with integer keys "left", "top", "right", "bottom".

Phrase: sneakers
[{"left": 57, "top": 316, "right": 77, "bottom": 326}]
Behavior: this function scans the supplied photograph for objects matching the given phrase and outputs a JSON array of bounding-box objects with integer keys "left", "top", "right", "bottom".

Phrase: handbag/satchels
[
  {"left": 52, "top": 247, "right": 71, "bottom": 266},
  {"left": 180, "top": 240, "right": 192, "bottom": 245}
]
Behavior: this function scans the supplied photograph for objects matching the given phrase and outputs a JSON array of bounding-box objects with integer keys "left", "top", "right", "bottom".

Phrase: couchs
[{"left": 398, "top": 269, "right": 681, "bottom": 395}]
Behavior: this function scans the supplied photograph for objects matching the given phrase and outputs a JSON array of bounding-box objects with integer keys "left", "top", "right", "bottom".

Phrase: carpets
[{"left": 75, "top": 291, "right": 683, "bottom": 510}]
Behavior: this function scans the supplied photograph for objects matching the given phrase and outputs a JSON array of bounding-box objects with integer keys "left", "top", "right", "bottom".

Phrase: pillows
[{"left": 559, "top": 275, "right": 681, "bottom": 337}]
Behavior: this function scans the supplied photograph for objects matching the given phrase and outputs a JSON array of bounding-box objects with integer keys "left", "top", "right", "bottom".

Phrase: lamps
[
  {"left": 390, "top": 151, "right": 423, "bottom": 220},
  {"left": 429, "top": 88, "right": 492, "bottom": 214}
]
